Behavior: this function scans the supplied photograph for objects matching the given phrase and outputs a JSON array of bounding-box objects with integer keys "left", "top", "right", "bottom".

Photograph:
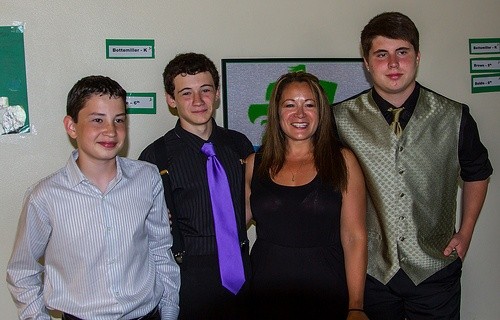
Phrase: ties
[
  {"left": 202, "top": 140, "right": 245, "bottom": 296},
  {"left": 388, "top": 107, "right": 405, "bottom": 140}
]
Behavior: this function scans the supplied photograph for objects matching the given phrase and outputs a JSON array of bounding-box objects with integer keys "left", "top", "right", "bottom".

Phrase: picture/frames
[{"left": 221, "top": 58, "right": 374, "bottom": 154}]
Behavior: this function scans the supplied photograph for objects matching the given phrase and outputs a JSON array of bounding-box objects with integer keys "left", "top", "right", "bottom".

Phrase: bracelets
[{"left": 348, "top": 308, "right": 365, "bottom": 312}]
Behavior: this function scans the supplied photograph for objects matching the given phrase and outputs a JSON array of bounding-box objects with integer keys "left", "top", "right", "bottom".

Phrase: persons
[
  {"left": 5, "top": 75, "right": 181, "bottom": 320},
  {"left": 138, "top": 53, "right": 255, "bottom": 320},
  {"left": 331, "top": 11, "right": 493, "bottom": 320},
  {"left": 243, "top": 71, "right": 367, "bottom": 320}
]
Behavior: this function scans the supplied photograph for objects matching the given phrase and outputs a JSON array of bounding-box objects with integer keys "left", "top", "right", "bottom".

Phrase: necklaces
[{"left": 284, "top": 159, "right": 310, "bottom": 184}]
